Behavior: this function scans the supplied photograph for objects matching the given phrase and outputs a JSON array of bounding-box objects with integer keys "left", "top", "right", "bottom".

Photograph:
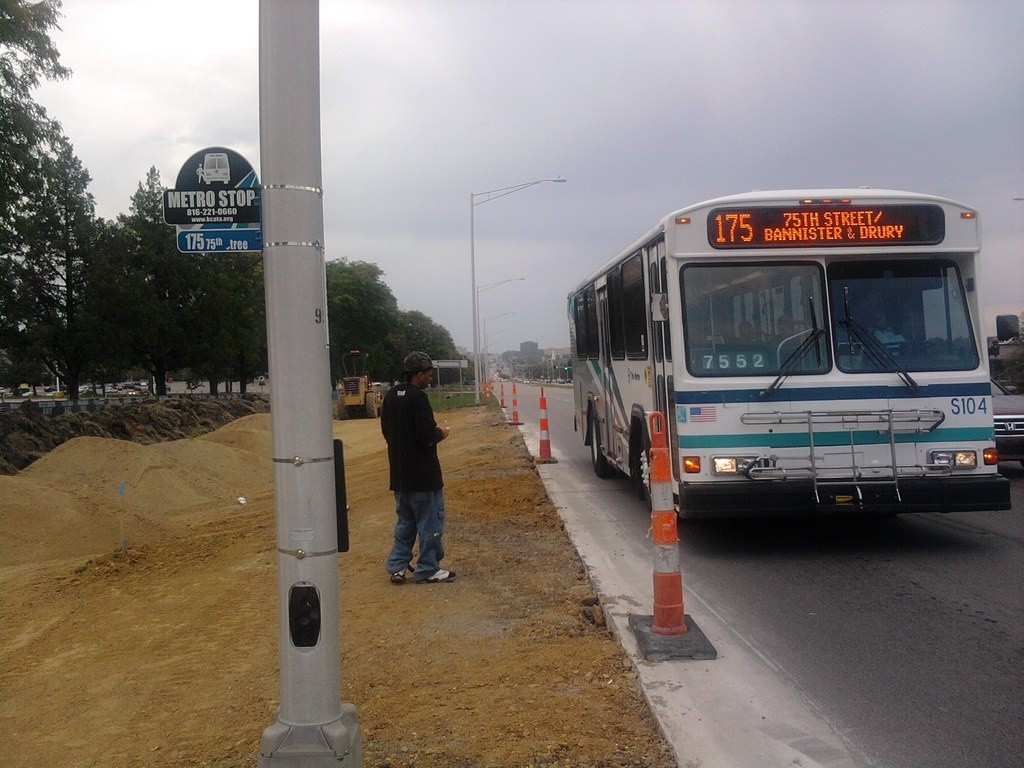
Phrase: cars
[
  {"left": 990, "top": 376, "right": 1024, "bottom": 468},
  {"left": 489, "top": 369, "right": 572, "bottom": 385},
  {"left": 22, "top": 381, "right": 151, "bottom": 396}
]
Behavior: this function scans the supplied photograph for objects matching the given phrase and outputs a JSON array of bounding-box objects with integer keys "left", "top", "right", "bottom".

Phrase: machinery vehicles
[{"left": 335, "top": 349, "right": 383, "bottom": 421}]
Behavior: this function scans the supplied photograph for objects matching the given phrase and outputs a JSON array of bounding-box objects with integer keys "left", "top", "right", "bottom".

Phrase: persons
[
  {"left": 769, "top": 315, "right": 801, "bottom": 344},
  {"left": 865, "top": 311, "right": 906, "bottom": 356},
  {"left": 381, "top": 351, "right": 457, "bottom": 584}
]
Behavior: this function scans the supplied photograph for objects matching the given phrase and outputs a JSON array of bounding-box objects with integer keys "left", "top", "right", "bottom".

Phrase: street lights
[
  {"left": 476, "top": 276, "right": 527, "bottom": 393},
  {"left": 470, "top": 177, "right": 567, "bottom": 403}
]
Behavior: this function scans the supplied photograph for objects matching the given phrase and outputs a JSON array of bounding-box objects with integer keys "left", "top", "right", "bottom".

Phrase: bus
[{"left": 566, "top": 186, "right": 1012, "bottom": 532}]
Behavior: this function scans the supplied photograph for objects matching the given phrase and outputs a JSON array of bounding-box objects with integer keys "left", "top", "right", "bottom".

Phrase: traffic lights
[{"left": 564, "top": 364, "right": 568, "bottom": 370}]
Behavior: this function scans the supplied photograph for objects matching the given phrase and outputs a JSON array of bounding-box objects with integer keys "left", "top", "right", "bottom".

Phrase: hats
[{"left": 403, "top": 351, "right": 433, "bottom": 371}]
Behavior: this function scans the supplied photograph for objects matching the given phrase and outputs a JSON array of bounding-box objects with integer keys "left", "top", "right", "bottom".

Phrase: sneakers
[
  {"left": 416, "top": 569, "right": 457, "bottom": 584},
  {"left": 390, "top": 567, "right": 407, "bottom": 583}
]
